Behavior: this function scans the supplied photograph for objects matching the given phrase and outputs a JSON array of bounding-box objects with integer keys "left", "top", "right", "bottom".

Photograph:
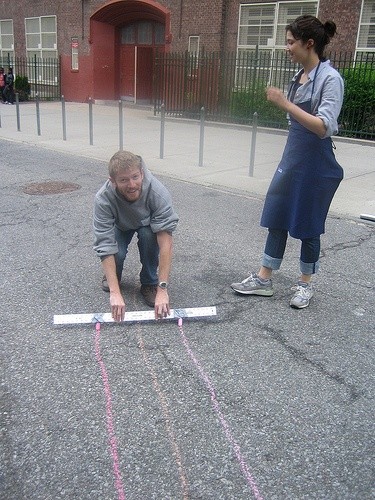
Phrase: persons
[
  {"left": 92, "top": 149, "right": 180, "bottom": 324},
  {"left": 230, "top": 15, "right": 344, "bottom": 309}
]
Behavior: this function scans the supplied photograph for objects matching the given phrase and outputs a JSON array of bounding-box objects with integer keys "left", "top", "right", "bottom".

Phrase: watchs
[{"left": 158, "top": 281, "right": 169, "bottom": 289}]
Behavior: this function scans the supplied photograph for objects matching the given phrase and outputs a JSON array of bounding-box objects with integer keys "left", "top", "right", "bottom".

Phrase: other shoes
[
  {"left": 141, "top": 284, "right": 157, "bottom": 307},
  {"left": 102, "top": 275, "right": 111, "bottom": 292}
]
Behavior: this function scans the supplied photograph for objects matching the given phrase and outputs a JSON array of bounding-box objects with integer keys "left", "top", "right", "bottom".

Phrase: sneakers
[
  {"left": 289, "top": 283, "right": 315, "bottom": 309},
  {"left": 230, "top": 271, "right": 274, "bottom": 297}
]
